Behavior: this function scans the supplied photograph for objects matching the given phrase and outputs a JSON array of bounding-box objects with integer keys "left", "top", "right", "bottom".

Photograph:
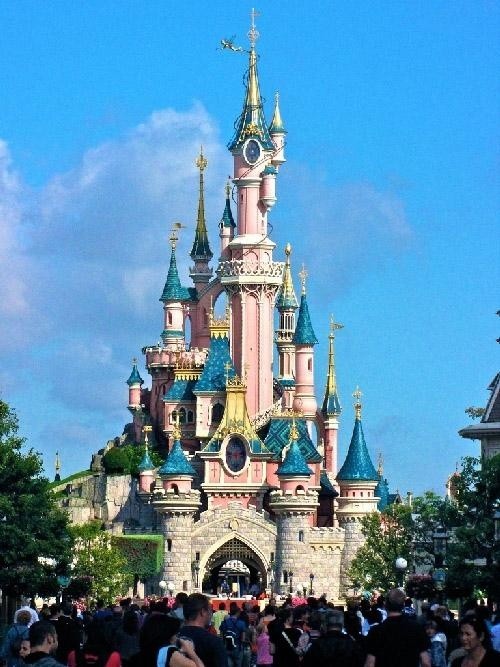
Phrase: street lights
[{"left": 432, "top": 525, "right": 446, "bottom": 599}]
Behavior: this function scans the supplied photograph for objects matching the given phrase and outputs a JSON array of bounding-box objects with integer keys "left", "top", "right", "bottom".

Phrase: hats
[{"left": 228, "top": 607, "right": 240, "bottom": 615}]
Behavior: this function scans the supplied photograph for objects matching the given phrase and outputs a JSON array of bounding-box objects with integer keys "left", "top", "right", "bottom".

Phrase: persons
[{"left": 0, "top": 578, "right": 500, "bottom": 667}]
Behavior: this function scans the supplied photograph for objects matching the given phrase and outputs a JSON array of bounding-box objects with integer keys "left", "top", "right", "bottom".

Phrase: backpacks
[
  {"left": 10, "top": 626, "right": 29, "bottom": 656},
  {"left": 222, "top": 619, "right": 240, "bottom": 650}
]
